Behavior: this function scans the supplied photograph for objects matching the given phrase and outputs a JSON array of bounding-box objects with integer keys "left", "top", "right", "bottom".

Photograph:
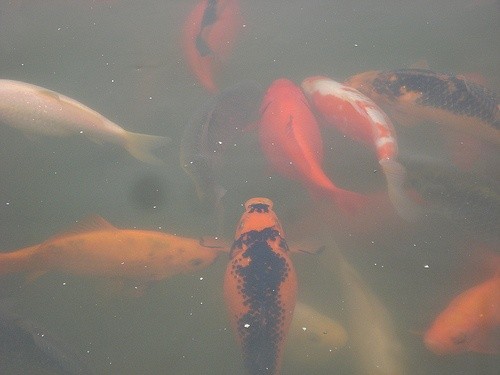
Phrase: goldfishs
[
  {"left": 200, "top": 197, "right": 318, "bottom": 375},
  {"left": 334, "top": 236, "right": 403, "bottom": 375},
  {"left": 180, "top": 0, "right": 244, "bottom": 95},
  {"left": 280, "top": 302, "right": 350, "bottom": 364},
  {"left": 411, "top": 253, "right": 500, "bottom": 360},
  {"left": 0, "top": 79, "right": 162, "bottom": 167},
  {"left": 257, "top": 78, "right": 366, "bottom": 215},
  {"left": 0, "top": 215, "right": 222, "bottom": 298},
  {"left": 299, "top": 76, "right": 418, "bottom": 222},
  {"left": 345, "top": 60, "right": 499, "bottom": 141}
]
